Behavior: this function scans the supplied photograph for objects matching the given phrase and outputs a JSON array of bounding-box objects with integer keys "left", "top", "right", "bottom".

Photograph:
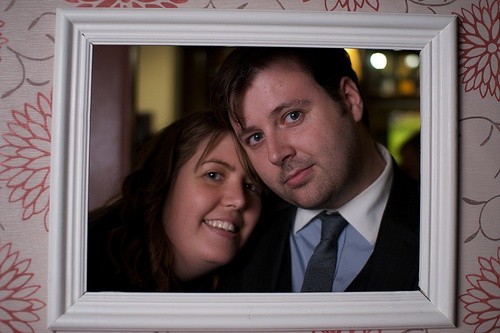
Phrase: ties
[{"left": 301, "top": 210, "right": 349, "bottom": 292}]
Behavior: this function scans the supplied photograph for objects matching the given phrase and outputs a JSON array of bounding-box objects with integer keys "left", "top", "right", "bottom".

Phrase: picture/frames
[{"left": 48, "top": 7, "right": 458, "bottom": 330}]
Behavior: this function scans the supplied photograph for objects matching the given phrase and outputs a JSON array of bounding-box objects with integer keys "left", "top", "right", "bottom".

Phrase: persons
[
  {"left": 399, "top": 131, "right": 420, "bottom": 180},
  {"left": 86, "top": 111, "right": 262, "bottom": 293},
  {"left": 209, "top": 46, "right": 421, "bottom": 292}
]
